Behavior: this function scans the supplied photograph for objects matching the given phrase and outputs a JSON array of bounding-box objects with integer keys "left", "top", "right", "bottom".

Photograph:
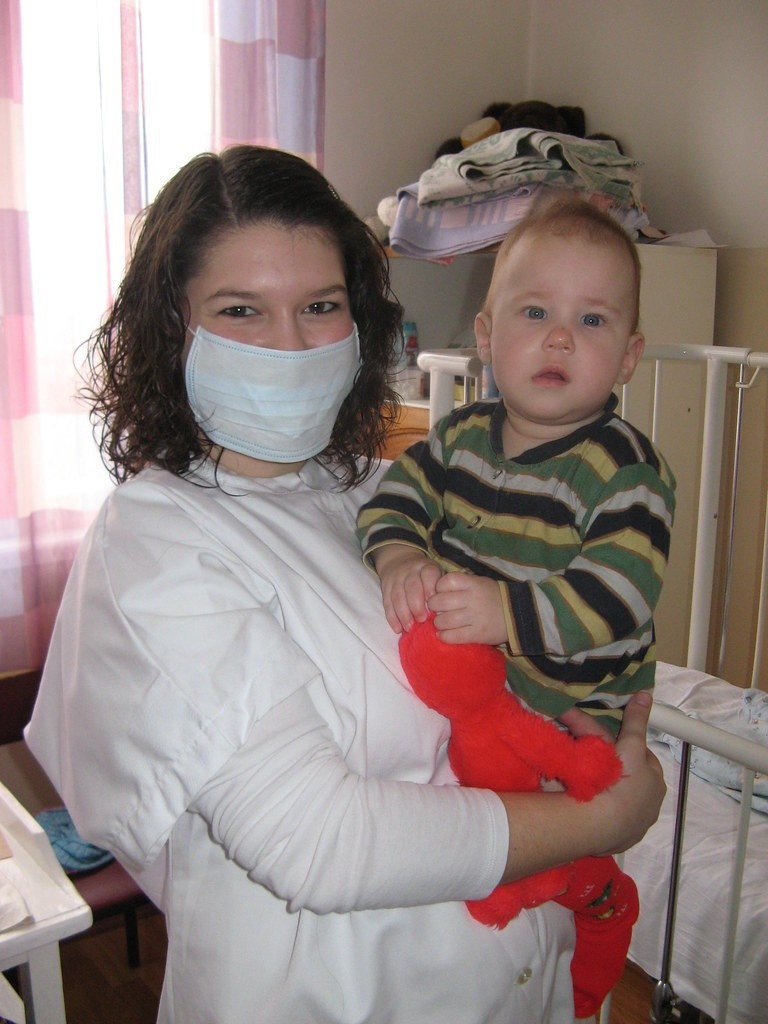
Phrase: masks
[{"left": 172, "top": 309, "right": 363, "bottom": 463}]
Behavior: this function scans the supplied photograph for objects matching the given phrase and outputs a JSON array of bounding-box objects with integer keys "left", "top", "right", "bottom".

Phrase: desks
[{"left": 0, "top": 782, "right": 94, "bottom": 1024}]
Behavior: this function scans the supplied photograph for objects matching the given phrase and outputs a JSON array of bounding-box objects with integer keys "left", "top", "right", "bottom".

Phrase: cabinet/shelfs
[
  {"left": 361, "top": 400, "right": 472, "bottom": 460},
  {"left": 613, "top": 244, "right": 719, "bottom": 667}
]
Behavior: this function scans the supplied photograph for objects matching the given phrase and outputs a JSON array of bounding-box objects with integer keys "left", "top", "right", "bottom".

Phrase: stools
[{"left": 1, "top": 740, "right": 161, "bottom": 974}]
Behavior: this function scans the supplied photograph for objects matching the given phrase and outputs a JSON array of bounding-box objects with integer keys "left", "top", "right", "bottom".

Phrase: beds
[{"left": 600, "top": 661, "right": 768, "bottom": 1024}]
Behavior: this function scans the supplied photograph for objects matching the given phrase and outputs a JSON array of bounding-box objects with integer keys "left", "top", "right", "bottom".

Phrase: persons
[
  {"left": 354, "top": 194, "right": 678, "bottom": 1019},
  {"left": 22, "top": 141, "right": 667, "bottom": 1024}
]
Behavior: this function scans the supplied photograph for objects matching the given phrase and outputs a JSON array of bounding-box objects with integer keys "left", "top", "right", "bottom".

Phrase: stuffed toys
[{"left": 396, "top": 610, "right": 639, "bottom": 933}]
